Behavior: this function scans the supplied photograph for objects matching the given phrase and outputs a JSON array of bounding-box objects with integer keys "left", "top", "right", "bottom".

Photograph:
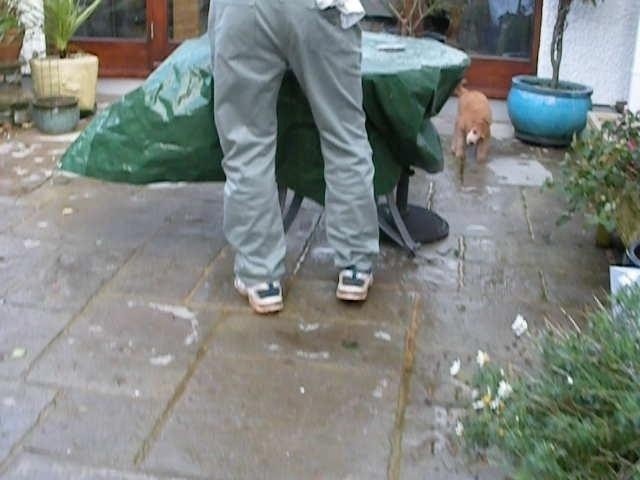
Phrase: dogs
[{"left": 450, "top": 78, "right": 491, "bottom": 164}]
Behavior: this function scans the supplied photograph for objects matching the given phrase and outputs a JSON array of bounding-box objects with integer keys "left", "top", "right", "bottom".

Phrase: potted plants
[
  {"left": 0, "top": 0, "right": 31, "bottom": 81},
  {"left": 19, "top": 0, "right": 111, "bottom": 117}
]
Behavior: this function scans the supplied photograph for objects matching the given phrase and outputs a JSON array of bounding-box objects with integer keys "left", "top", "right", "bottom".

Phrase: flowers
[{"left": 557, "top": 102, "right": 640, "bottom": 236}]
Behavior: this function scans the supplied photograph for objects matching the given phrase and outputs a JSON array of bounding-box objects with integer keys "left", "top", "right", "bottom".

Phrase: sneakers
[
  {"left": 233, "top": 273, "right": 283, "bottom": 314},
  {"left": 335, "top": 267, "right": 373, "bottom": 301}
]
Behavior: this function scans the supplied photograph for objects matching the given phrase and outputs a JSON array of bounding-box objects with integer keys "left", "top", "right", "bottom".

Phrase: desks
[{"left": 59, "top": 24, "right": 470, "bottom": 259}]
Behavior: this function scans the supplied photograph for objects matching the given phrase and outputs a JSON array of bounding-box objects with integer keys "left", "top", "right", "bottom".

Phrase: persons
[{"left": 207, "top": 0, "right": 380, "bottom": 313}]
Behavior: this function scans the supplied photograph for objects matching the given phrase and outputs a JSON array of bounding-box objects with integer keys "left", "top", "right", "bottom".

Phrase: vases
[
  {"left": 29, "top": 94, "right": 81, "bottom": 136},
  {"left": 621, "top": 236, "right": 640, "bottom": 268},
  {"left": 507, "top": 74, "right": 593, "bottom": 151}
]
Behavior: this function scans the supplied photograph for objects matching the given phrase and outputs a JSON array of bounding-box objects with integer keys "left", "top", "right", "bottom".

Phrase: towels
[{"left": 317, "top": 0, "right": 365, "bottom": 29}]
